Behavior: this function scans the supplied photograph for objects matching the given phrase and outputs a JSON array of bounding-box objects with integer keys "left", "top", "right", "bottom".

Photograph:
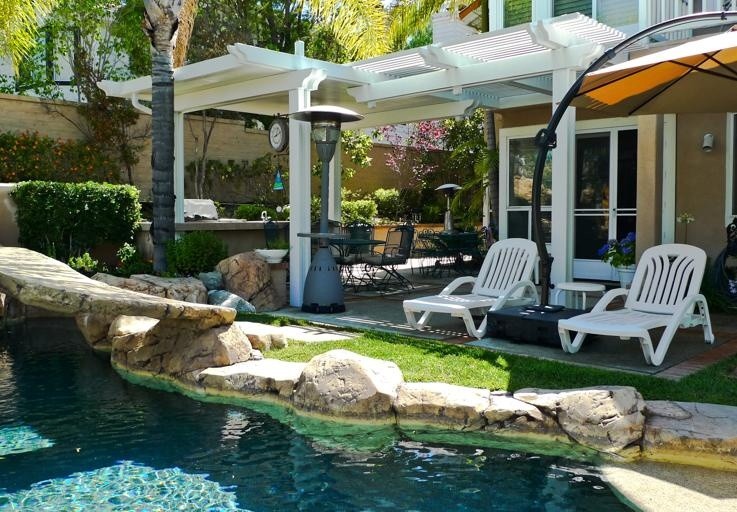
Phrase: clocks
[{"left": 269, "top": 114, "right": 287, "bottom": 190}]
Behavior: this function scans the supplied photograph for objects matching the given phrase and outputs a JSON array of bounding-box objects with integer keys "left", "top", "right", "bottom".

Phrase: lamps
[{"left": 702, "top": 133, "right": 714, "bottom": 153}]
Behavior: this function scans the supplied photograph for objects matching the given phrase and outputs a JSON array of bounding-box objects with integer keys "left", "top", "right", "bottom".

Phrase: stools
[{"left": 553, "top": 281, "right": 607, "bottom": 312}]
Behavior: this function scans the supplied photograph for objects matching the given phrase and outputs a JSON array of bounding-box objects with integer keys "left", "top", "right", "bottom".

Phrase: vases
[{"left": 617, "top": 268, "right": 640, "bottom": 293}]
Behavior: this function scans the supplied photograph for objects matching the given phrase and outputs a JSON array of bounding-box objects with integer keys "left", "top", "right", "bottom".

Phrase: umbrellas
[{"left": 530, "top": 10, "right": 737, "bottom": 306}]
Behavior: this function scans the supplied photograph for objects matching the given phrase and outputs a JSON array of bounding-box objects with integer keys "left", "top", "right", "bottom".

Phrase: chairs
[
  {"left": 401, "top": 237, "right": 540, "bottom": 338},
  {"left": 557, "top": 242, "right": 716, "bottom": 366},
  {"left": 315, "top": 219, "right": 494, "bottom": 297}
]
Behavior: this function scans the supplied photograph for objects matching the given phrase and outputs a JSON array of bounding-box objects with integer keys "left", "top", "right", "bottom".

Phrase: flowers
[{"left": 598, "top": 230, "right": 633, "bottom": 266}]
